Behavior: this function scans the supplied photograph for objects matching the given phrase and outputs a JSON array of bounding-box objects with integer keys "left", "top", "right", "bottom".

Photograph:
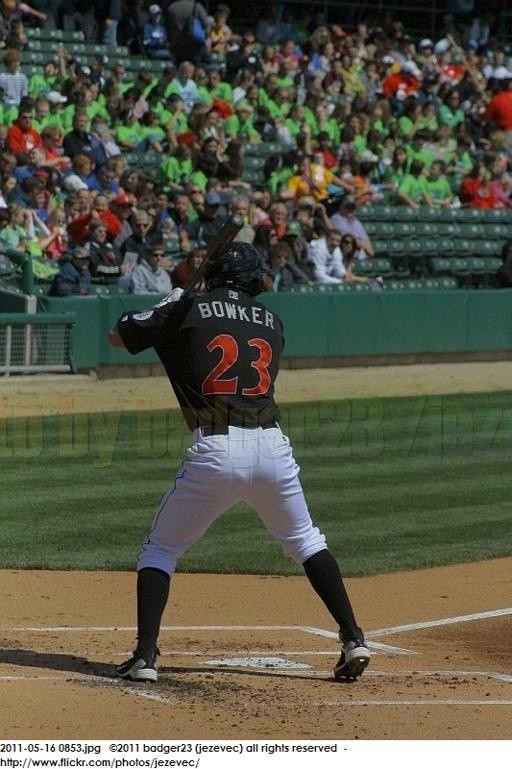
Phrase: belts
[{"left": 202, "top": 422, "right": 277, "bottom": 438}]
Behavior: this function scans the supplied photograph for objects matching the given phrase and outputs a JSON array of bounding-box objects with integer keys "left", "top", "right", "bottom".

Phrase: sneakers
[
  {"left": 333, "top": 639, "right": 371, "bottom": 680},
  {"left": 116, "top": 650, "right": 161, "bottom": 682}
]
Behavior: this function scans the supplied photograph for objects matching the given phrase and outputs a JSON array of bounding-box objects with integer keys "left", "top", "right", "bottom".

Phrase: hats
[
  {"left": 205, "top": 192, "right": 221, "bottom": 206},
  {"left": 403, "top": 60, "right": 420, "bottom": 76},
  {"left": 47, "top": 92, "right": 67, "bottom": 103},
  {"left": 149, "top": 4, "right": 160, "bottom": 14},
  {"left": 285, "top": 221, "right": 301, "bottom": 237},
  {"left": 298, "top": 195, "right": 315, "bottom": 209}
]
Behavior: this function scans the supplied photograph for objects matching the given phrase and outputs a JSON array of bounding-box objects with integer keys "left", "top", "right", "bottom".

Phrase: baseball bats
[{"left": 187, "top": 215, "right": 243, "bottom": 289}]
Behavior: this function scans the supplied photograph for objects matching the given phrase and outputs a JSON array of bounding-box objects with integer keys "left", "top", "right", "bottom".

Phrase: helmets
[{"left": 205, "top": 241, "right": 274, "bottom": 297}]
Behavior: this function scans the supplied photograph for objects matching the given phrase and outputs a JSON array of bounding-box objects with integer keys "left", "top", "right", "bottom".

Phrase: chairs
[{"left": 1, "top": 26, "right": 512, "bottom": 296}]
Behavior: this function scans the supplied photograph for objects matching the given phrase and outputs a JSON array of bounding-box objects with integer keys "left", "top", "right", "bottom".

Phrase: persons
[
  {"left": 1, "top": 0, "right": 511, "bottom": 294},
  {"left": 108, "top": 240, "right": 370, "bottom": 682}
]
